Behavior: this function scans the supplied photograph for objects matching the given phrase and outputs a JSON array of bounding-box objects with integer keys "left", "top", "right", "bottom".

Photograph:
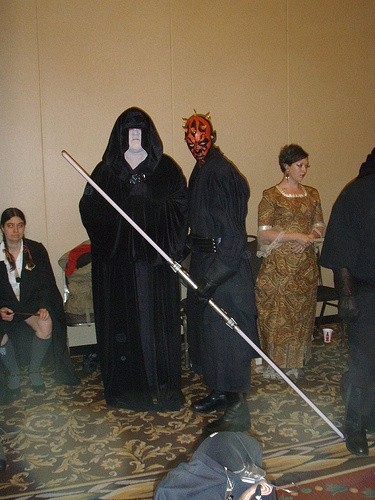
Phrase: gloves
[{"left": 190, "top": 279, "right": 215, "bottom": 311}]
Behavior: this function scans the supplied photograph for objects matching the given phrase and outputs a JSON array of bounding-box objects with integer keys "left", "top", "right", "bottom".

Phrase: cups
[{"left": 323, "top": 329, "right": 333, "bottom": 343}]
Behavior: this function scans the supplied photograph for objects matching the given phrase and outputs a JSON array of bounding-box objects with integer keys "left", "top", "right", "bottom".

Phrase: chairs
[
  {"left": 52, "top": 236, "right": 189, "bottom": 373},
  {"left": 311, "top": 247, "right": 340, "bottom": 327}
]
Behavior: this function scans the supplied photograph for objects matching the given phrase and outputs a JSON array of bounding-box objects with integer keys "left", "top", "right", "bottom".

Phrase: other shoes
[
  {"left": 28, "top": 370, "right": 45, "bottom": 393},
  {"left": 5, "top": 374, "right": 21, "bottom": 398}
]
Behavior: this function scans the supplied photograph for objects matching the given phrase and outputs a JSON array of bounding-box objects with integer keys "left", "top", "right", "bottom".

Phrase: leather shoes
[
  {"left": 190, "top": 390, "right": 225, "bottom": 412},
  {"left": 346, "top": 431, "right": 369, "bottom": 455},
  {"left": 204, "top": 400, "right": 251, "bottom": 433}
]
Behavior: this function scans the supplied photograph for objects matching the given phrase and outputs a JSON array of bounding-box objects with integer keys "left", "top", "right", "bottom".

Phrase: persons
[
  {"left": 319, "top": 147, "right": 375, "bottom": 457},
  {"left": 0, "top": 207, "right": 65, "bottom": 396},
  {"left": 77, "top": 106, "right": 190, "bottom": 412},
  {"left": 52, "top": 240, "right": 97, "bottom": 387},
  {"left": 257, "top": 143, "right": 326, "bottom": 382},
  {"left": 178, "top": 107, "right": 261, "bottom": 431}
]
